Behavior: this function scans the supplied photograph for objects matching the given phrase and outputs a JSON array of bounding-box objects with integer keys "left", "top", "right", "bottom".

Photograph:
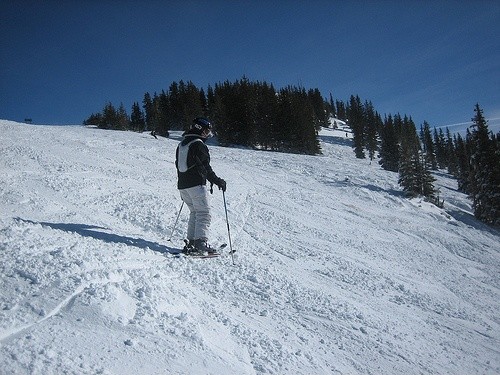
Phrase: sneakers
[
  {"left": 183, "top": 238, "right": 196, "bottom": 253},
  {"left": 192, "top": 239, "right": 217, "bottom": 255}
]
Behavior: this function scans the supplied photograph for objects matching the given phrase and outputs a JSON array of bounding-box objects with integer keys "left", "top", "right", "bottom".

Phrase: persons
[{"left": 175, "top": 118, "right": 226, "bottom": 256}]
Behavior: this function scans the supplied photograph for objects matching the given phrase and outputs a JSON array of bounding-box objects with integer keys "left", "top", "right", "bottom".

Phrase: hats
[{"left": 191, "top": 117, "right": 213, "bottom": 136}]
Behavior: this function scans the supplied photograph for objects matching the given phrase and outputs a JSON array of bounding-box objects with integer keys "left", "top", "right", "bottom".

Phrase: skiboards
[
  {"left": 174, "top": 249, "right": 236, "bottom": 258},
  {"left": 171, "top": 243, "right": 228, "bottom": 255}
]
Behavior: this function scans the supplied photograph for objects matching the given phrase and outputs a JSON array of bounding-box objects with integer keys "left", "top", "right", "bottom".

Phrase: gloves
[{"left": 216, "top": 178, "right": 227, "bottom": 192}]
